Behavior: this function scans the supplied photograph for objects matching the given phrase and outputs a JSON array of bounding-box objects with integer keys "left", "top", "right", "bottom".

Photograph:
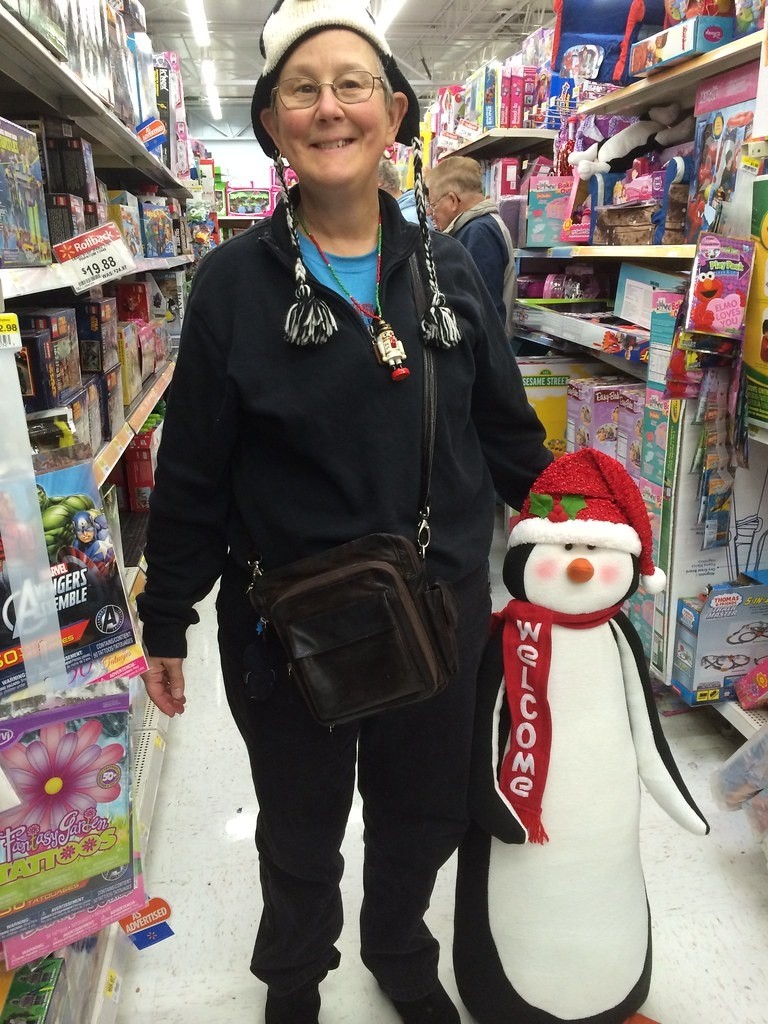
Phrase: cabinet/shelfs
[{"left": 0, "top": 0, "right": 768, "bottom": 1024}]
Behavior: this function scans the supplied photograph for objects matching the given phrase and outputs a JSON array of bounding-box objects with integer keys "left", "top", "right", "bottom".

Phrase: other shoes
[
  {"left": 391, "top": 982, "right": 461, "bottom": 1024},
  {"left": 265, "top": 986, "right": 321, "bottom": 1024}
]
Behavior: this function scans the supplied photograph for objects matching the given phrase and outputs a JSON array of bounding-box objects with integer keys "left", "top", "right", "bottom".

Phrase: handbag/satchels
[{"left": 251, "top": 533, "right": 460, "bottom": 726}]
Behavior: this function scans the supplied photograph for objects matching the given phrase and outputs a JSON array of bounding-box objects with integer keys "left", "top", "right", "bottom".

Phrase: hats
[{"left": 250, "top": 0, "right": 463, "bottom": 350}]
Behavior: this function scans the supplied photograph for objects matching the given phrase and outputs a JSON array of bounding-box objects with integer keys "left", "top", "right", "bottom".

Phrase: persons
[
  {"left": 136, "top": 0, "right": 558, "bottom": 1024},
  {"left": 720, "top": 150, "right": 734, "bottom": 187},
  {"left": 562, "top": 47, "right": 592, "bottom": 78}
]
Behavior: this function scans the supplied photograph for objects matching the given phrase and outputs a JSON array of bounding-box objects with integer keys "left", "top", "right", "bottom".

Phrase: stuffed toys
[
  {"left": 452, "top": 448, "right": 710, "bottom": 1024},
  {"left": 567, "top": 102, "right": 695, "bottom": 182}
]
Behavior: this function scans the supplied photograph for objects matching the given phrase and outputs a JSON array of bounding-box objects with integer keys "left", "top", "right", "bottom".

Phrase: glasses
[
  {"left": 426, "top": 192, "right": 461, "bottom": 211},
  {"left": 270, "top": 71, "right": 387, "bottom": 111}
]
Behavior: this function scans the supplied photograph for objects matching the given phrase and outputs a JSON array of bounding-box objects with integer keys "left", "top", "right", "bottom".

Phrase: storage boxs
[
  {"left": 504, "top": 264, "right": 768, "bottom": 708},
  {"left": 629, "top": 17, "right": 735, "bottom": 79},
  {"left": 23, "top": 296, "right": 125, "bottom": 457},
  {"left": 128, "top": 417, "right": 163, "bottom": 514},
  {"left": 215, "top": 167, "right": 283, "bottom": 216},
  {"left": 518, "top": 176, "right": 581, "bottom": 246}
]
